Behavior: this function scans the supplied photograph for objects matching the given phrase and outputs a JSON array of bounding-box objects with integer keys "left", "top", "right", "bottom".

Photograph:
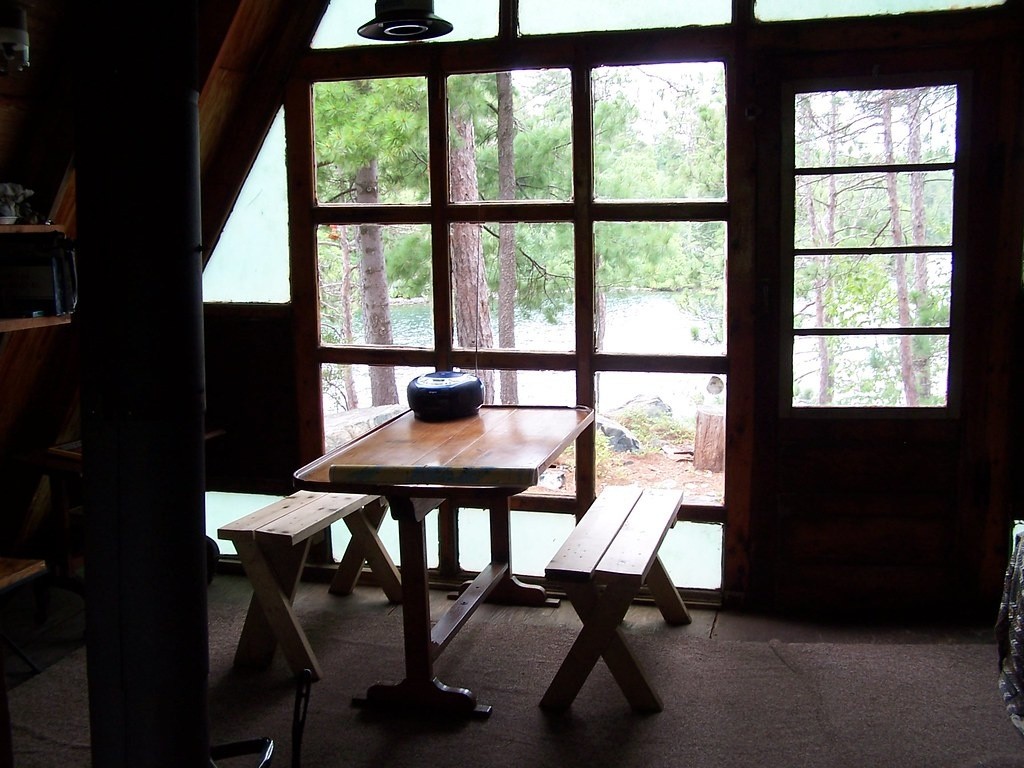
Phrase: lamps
[{"left": 357, "top": 0, "right": 454, "bottom": 41}]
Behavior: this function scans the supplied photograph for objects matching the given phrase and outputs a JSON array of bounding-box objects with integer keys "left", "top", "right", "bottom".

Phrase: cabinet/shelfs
[{"left": 0, "top": 226, "right": 80, "bottom": 590}]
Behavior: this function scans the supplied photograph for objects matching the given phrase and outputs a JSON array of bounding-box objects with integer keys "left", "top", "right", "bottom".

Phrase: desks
[{"left": 291, "top": 403, "right": 597, "bottom": 721}]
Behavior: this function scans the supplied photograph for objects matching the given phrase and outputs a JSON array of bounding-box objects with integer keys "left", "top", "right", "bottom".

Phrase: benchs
[
  {"left": 536, "top": 490, "right": 694, "bottom": 718},
  {"left": 216, "top": 490, "right": 402, "bottom": 680}
]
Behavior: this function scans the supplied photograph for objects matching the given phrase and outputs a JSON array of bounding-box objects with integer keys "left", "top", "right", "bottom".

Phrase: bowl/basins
[{"left": 0, "top": 216, "right": 19, "bottom": 225}]
